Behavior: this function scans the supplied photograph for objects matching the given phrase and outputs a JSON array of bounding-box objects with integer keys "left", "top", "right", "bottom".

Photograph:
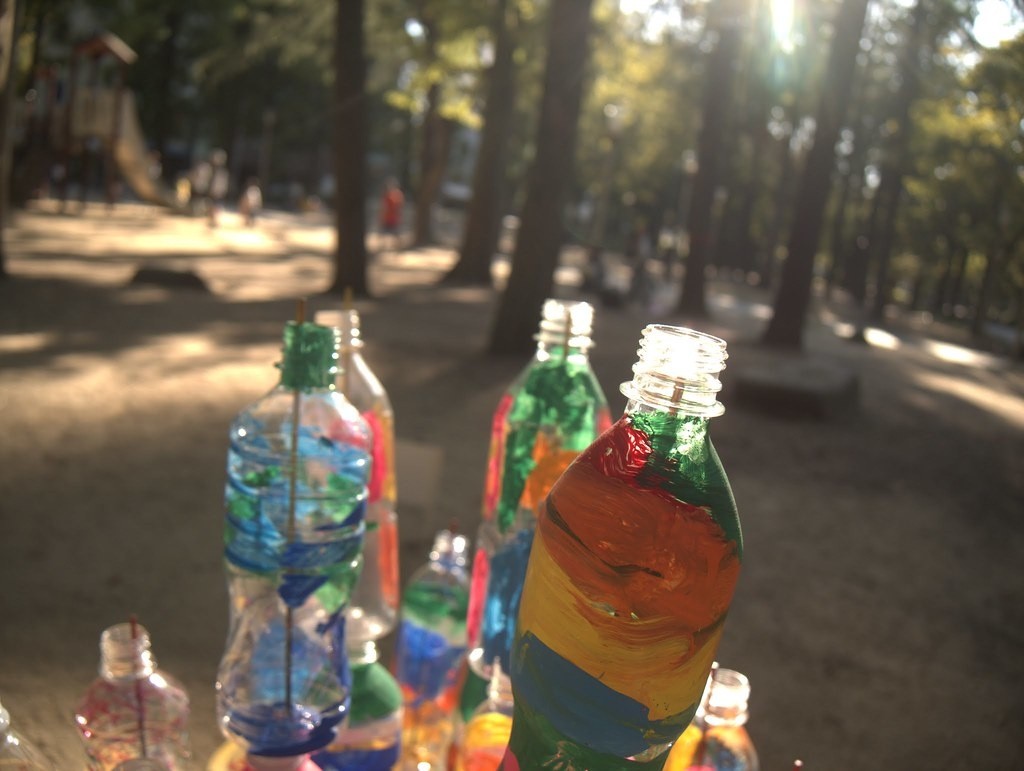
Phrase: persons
[{"left": 377, "top": 175, "right": 406, "bottom": 239}]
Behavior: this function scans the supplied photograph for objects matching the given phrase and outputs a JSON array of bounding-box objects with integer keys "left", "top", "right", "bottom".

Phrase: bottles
[{"left": 1, "top": 299, "right": 759, "bottom": 770}]
